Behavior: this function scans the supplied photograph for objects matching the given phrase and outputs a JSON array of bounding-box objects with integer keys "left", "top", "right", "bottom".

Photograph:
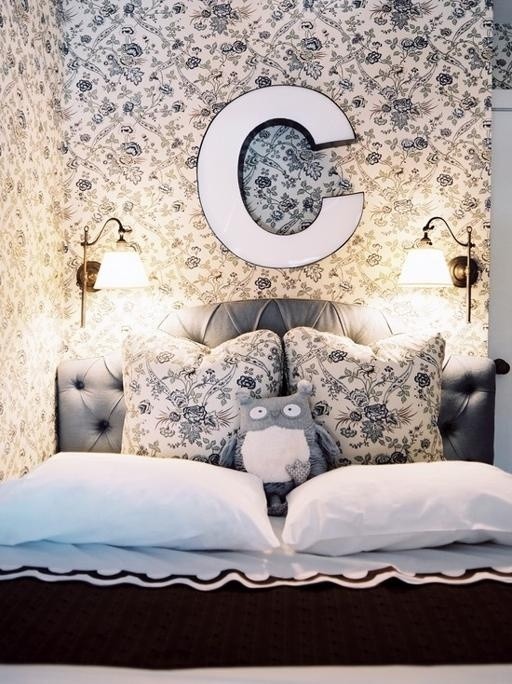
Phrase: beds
[{"left": 0, "top": 297, "right": 512, "bottom": 672}]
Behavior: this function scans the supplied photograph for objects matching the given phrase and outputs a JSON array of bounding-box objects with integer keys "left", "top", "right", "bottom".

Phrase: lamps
[
  {"left": 396, "top": 213, "right": 483, "bottom": 325},
  {"left": 77, "top": 216, "right": 150, "bottom": 330}
]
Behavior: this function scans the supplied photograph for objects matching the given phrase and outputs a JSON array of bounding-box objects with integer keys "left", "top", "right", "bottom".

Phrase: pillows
[
  {"left": 283, "top": 326, "right": 447, "bottom": 465},
  {"left": 281, "top": 460, "right": 512, "bottom": 555},
  {"left": 120, "top": 330, "right": 282, "bottom": 468},
  {"left": 1, "top": 452, "right": 280, "bottom": 554}
]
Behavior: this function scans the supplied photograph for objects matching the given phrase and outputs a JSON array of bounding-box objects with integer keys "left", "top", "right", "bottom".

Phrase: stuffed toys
[{"left": 216, "top": 380, "right": 342, "bottom": 516}]
